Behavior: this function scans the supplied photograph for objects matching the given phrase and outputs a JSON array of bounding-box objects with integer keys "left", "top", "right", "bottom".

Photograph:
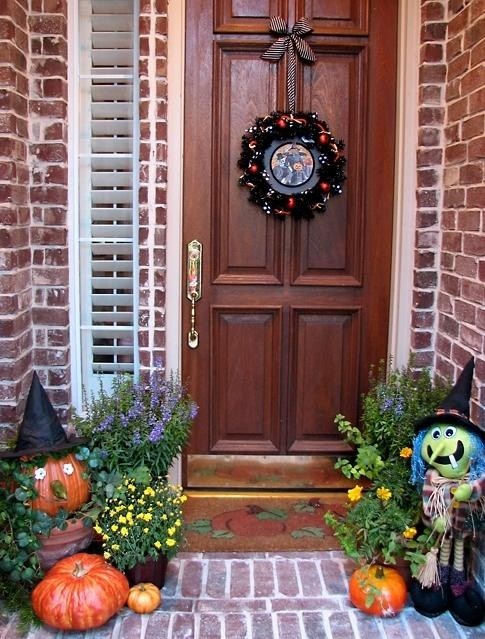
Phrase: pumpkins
[
  {"left": 128, "top": 584, "right": 160, "bottom": 615},
  {"left": 350, "top": 563, "right": 407, "bottom": 618},
  {"left": 32, "top": 550, "right": 128, "bottom": 630},
  {"left": 0, "top": 453, "right": 89, "bottom": 514}
]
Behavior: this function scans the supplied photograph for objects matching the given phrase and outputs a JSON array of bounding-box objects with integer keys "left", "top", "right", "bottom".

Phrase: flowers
[
  {"left": 320, "top": 347, "right": 460, "bottom": 563},
  {"left": 68, "top": 353, "right": 202, "bottom": 556}
]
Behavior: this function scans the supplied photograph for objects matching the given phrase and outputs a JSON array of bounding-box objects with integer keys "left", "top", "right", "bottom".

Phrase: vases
[
  {"left": 378, "top": 557, "right": 414, "bottom": 592},
  {"left": 123, "top": 556, "right": 166, "bottom": 596}
]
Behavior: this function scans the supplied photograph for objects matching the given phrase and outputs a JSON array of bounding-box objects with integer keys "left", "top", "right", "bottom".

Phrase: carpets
[{"left": 165, "top": 491, "right": 390, "bottom": 555}]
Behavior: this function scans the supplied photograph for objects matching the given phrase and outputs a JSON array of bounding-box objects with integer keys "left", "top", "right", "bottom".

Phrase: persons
[{"left": 409, "top": 353, "right": 485, "bottom": 627}]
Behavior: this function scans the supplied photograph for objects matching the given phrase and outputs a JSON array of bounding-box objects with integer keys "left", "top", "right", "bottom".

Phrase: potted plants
[{"left": 0, "top": 439, "right": 94, "bottom": 574}]
